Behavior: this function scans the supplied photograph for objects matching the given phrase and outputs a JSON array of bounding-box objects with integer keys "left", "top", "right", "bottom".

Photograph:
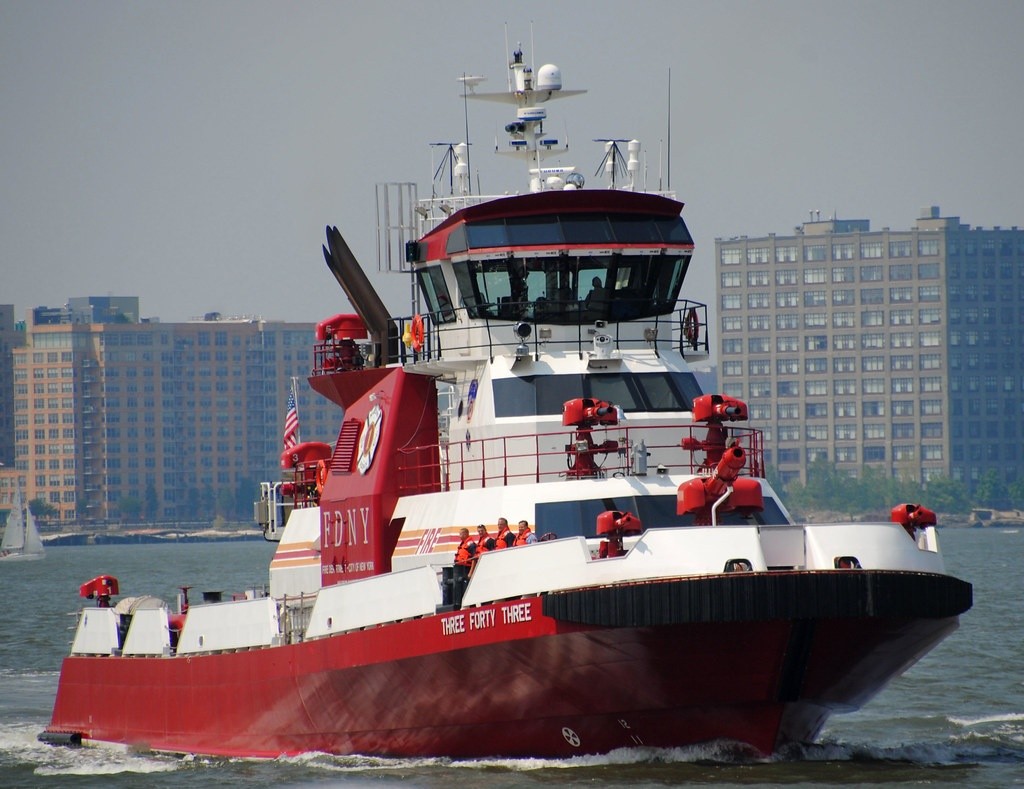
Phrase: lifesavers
[
  {"left": 314, "top": 459, "right": 327, "bottom": 496},
  {"left": 410, "top": 313, "right": 425, "bottom": 353},
  {"left": 684, "top": 312, "right": 699, "bottom": 340}
]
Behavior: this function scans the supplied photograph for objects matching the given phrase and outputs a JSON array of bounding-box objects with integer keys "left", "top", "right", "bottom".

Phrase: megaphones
[{"left": 595, "top": 320, "right": 608, "bottom": 329}]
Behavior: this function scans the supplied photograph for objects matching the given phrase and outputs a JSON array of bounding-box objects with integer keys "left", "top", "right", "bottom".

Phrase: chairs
[{"left": 497, "top": 296, "right": 512, "bottom": 319}]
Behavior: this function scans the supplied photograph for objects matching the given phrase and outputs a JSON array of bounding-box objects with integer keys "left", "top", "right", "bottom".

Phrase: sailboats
[{"left": 0, "top": 485, "right": 45, "bottom": 562}]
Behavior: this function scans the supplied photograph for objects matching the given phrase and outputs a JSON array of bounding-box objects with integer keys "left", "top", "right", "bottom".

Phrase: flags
[{"left": 284, "top": 388, "right": 299, "bottom": 451}]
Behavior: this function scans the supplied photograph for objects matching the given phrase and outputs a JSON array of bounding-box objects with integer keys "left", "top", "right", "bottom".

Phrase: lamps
[
  {"left": 513, "top": 321, "right": 531, "bottom": 356},
  {"left": 414, "top": 207, "right": 428, "bottom": 220},
  {"left": 438, "top": 204, "right": 452, "bottom": 216},
  {"left": 575, "top": 439, "right": 588, "bottom": 452}
]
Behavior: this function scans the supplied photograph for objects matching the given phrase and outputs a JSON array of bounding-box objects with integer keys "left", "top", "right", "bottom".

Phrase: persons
[
  {"left": 585, "top": 277, "right": 604, "bottom": 301},
  {"left": 513, "top": 521, "right": 537, "bottom": 547},
  {"left": 454, "top": 529, "right": 476, "bottom": 608},
  {"left": 475, "top": 525, "right": 496, "bottom": 605},
  {"left": 494, "top": 518, "right": 522, "bottom": 601}
]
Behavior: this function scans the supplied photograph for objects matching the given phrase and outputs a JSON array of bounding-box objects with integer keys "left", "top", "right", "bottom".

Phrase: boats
[{"left": 40, "top": 21, "right": 973, "bottom": 760}]
